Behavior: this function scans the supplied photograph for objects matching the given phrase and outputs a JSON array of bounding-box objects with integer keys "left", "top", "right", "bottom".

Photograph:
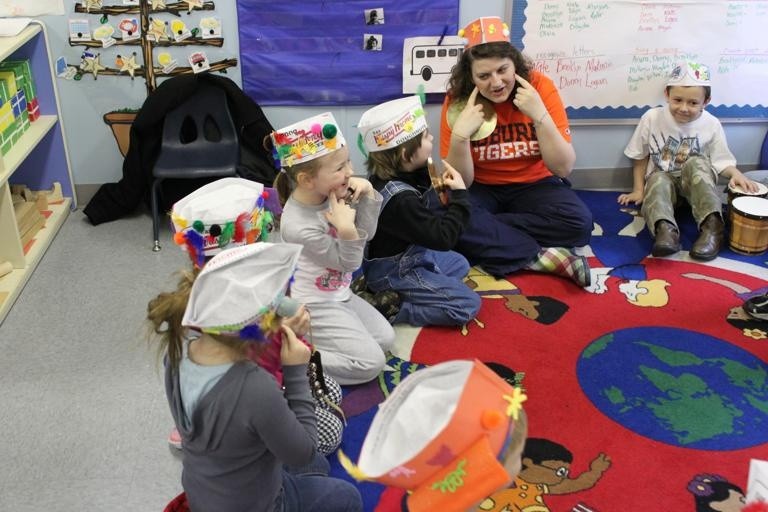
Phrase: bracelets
[{"left": 531, "top": 112, "right": 546, "bottom": 127}]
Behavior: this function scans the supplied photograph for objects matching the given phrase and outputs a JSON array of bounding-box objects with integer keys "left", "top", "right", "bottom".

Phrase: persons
[
  {"left": 350, "top": 95, "right": 481, "bottom": 328},
  {"left": 144, "top": 239, "right": 363, "bottom": 512},
  {"left": 269, "top": 112, "right": 397, "bottom": 385},
  {"left": 366, "top": 39, "right": 378, "bottom": 50},
  {"left": 431, "top": 17, "right": 594, "bottom": 288},
  {"left": 616, "top": 63, "right": 760, "bottom": 260},
  {"left": 164, "top": 177, "right": 343, "bottom": 458}
]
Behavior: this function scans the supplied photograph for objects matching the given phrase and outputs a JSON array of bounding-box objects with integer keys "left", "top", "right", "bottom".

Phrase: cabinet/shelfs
[{"left": 1, "top": 20, "right": 76, "bottom": 326}]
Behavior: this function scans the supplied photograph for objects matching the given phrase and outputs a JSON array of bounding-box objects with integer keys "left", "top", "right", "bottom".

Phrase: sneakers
[
  {"left": 351, "top": 274, "right": 400, "bottom": 319},
  {"left": 743, "top": 295, "right": 768, "bottom": 320}
]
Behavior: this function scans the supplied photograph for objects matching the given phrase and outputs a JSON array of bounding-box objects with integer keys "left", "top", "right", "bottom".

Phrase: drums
[
  {"left": 728, "top": 180, "right": 768, "bottom": 225},
  {"left": 729, "top": 196, "right": 768, "bottom": 256}
]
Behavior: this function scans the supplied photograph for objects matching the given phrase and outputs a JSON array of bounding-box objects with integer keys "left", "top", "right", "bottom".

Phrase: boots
[
  {"left": 523, "top": 247, "right": 590, "bottom": 287},
  {"left": 689, "top": 214, "right": 725, "bottom": 262},
  {"left": 652, "top": 221, "right": 680, "bottom": 256}
]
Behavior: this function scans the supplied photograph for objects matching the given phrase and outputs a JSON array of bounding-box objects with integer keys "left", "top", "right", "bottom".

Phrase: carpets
[{"left": 324, "top": 185, "right": 767, "bottom": 512}]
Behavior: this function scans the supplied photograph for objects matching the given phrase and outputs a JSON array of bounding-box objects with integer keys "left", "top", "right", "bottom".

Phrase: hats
[
  {"left": 171, "top": 177, "right": 282, "bottom": 271},
  {"left": 358, "top": 359, "right": 527, "bottom": 512},
  {"left": 269, "top": 111, "right": 346, "bottom": 170},
  {"left": 181, "top": 242, "right": 303, "bottom": 345},
  {"left": 458, "top": 16, "right": 510, "bottom": 53},
  {"left": 357, "top": 85, "right": 428, "bottom": 158},
  {"left": 667, "top": 61, "right": 711, "bottom": 87}
]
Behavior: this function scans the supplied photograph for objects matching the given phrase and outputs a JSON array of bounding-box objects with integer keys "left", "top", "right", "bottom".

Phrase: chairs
[{"left": 149, "top": 86, "right": 242, "bottom": 252}]
